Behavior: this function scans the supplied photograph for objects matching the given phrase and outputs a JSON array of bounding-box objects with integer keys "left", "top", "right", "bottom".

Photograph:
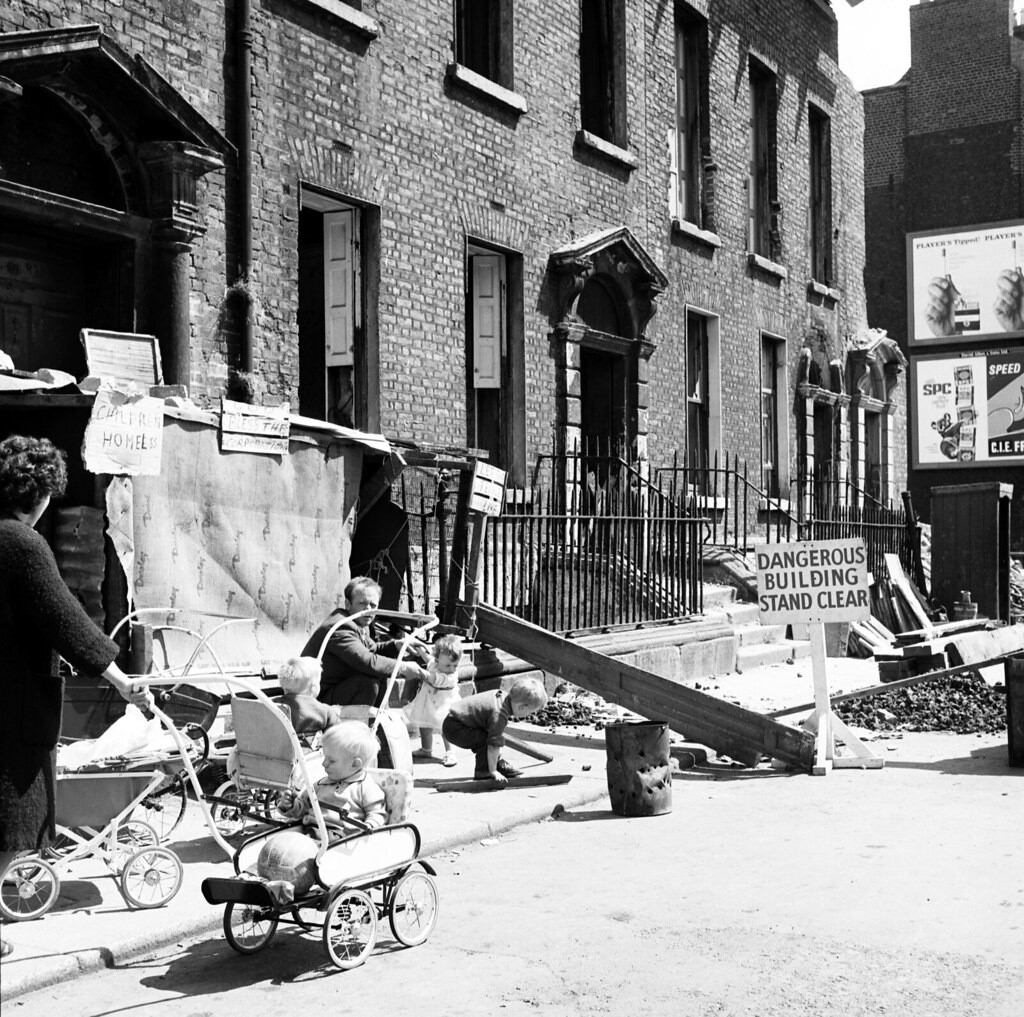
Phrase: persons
[
  {"left": 0, "top": 434, "right": 159, "bottom": 955},
  {"left": 234, "top": 715, "right": 390, "bottom": 893},
  {"left": 442, "top": 676, "right": 549, "bottom": 783},
  {"left": 993, "top": 266, "right": 1024, "bottom": 332},
  {"left": 271, "top": 655, "right": 344, "bottom": 756},
  {"left": 402, "top": 635, "right": 466, "bottom": 766},
  {"left": 301, "top": 575, "right": 430, "bottom": 725},
  {"left": 923, "top": 273, "right": 966, "bottom": 336}
]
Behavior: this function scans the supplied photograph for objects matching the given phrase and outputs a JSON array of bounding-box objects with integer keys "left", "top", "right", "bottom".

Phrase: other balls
[{"left": 256, "top": 831, "right": 320, "bottom": 894}]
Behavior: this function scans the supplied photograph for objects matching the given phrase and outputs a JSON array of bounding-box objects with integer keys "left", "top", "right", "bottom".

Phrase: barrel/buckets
[
  {"left": 1004, "top": 656, "right": 1024, "bottom": 768},
  {"left": 603, "top": 720, "right": 672, "bottom": 817}
]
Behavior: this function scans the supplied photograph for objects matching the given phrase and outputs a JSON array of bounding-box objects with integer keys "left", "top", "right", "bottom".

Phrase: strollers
[
  {"left": 139, "top": 610, "right": 437, "bottom": 971},
  {"left": 1, "top": 605, "right": 256, "bottom": 921}
]
